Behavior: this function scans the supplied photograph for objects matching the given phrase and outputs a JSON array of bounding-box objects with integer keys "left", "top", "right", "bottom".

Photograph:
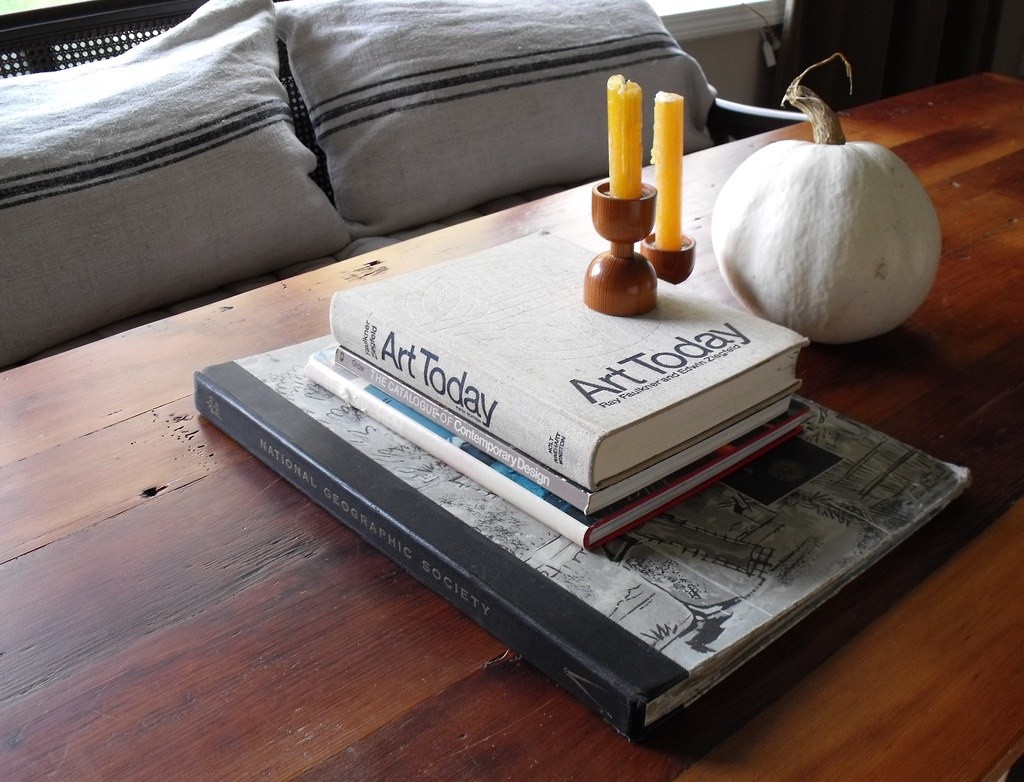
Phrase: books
[
  {"left": 328, "top": 228, "right": 811, "bottom": 494},
  {"left": 302, "top": 343, "right": 814, "bottom": 552},
  {"left": 194, "top": 335, "right": 975, "bottom": 744},
  {"left": 330, "top": 343, "right": 802, "bottom": 515}
]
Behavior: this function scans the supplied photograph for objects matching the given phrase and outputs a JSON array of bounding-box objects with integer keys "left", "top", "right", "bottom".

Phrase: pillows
[
  {"left": 0, "top": 0, "right": 352, "bottom": 371},
  {"left": 274, "top": 0, "right": 719, "bottom": 239}
]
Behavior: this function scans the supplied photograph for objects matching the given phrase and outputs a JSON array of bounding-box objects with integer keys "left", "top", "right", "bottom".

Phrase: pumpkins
[{"left": 709, "top": 80, "right": 941, "bottom": 344}]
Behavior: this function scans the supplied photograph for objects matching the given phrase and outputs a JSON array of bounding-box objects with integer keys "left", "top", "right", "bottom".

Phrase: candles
[
  {"left": 606, "top": 75, "right": 644, "bottom": 199},
  {"left": 650, "top": 92, "right": 683, "bottom": 252}
]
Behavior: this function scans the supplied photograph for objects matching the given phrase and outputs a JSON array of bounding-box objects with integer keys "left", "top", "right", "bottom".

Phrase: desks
[{"left": 0, "top": 72, "right": 1024, "bottom": 782}]
